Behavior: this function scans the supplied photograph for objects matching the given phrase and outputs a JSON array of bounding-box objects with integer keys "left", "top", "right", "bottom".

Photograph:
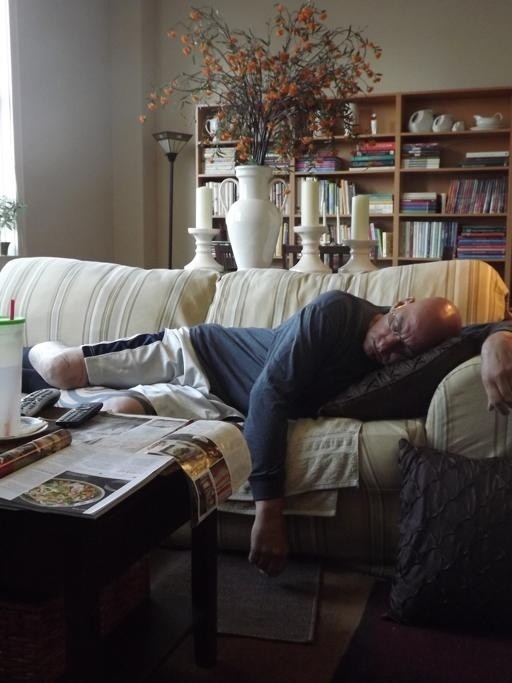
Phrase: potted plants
[{"left": 0, "top": 196, "right": 27, "bottom": 256}]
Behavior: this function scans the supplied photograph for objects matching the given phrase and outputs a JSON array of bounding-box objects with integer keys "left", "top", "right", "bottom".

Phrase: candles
[
  {"left": 301, "top": 182, "right": 320, "bottom": 227},
  {"left": 195, "top": 187, "right": 212, "bottom": 228},
  {"left": 350, "top": 196, "right": 370, "bottom": 240}
]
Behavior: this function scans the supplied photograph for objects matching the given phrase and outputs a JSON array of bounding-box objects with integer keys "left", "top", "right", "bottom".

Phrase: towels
[{"left": 217, "top": 417, "right": 362, "bottom": 517}]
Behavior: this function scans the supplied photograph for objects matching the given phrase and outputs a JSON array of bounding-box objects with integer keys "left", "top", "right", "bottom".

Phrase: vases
[{"left": 225, "top": 165, "right": 282, "bottom": 270}]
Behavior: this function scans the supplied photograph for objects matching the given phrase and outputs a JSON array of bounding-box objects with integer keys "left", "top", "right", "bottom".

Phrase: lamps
[{"left": 151, "top": 132, "right": 193, "bottom": 268}]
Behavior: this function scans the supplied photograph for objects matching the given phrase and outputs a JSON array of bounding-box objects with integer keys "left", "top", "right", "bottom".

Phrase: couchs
[{"left": 0, "top": 257, "right": 512, "bottom": 563}]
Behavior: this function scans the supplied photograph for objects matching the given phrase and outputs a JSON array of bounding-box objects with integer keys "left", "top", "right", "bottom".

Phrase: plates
[
  {"left": 21, "top": 477, "right": 106, "bottom": 508},
  {"left": 21, "top": 415, "right": 48, "bottom": 438}
]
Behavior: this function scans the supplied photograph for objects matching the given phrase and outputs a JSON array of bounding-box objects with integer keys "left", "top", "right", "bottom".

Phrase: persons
[{"left": 23, "top": 288, "right": 511, "bottom": 572}]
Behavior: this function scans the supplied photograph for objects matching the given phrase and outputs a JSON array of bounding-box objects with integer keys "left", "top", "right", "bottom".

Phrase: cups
[{"left": 0, "top": 316, "right": 27, "bottom": 437}]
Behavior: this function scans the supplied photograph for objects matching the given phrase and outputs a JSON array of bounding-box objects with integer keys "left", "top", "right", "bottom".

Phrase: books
[
  {"left": 204, "top": 147, "right": 290, "bottom": 258},
  {"left": 66, "top": 409, "right": 253, "bottom": 531},
  {"left": 0, "top": 427, "right": 176, "bottom": 520},
  {"left": 399, "top": 144, "right": 509, "bottom": 261},
  {"left": 296, "top": 142, "right": 396, "bottom": 259}
]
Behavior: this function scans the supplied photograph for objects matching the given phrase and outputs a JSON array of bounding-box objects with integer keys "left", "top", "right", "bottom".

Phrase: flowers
[{"left": 138, "top": 0, "right": 383, "bottom": 173}]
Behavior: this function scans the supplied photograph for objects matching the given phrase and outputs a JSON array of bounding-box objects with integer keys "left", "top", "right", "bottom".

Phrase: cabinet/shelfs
[
  {"left": 396, "top": 87, "right": 512, "bottom": 289},
  {"left": 195, "top": 92, "right": 398, "bottom": 271}
]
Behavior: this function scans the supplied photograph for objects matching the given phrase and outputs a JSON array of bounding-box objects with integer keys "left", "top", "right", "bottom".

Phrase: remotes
[
  {"left": 20, "top": 388, "right": 61, "bottom": 417},
  {"left": 55, "top": 402, "right": 103, "bottom": 427}
]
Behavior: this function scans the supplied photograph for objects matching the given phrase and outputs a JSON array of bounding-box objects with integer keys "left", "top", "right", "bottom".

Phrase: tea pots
[
  {"left": 473, "top": 112, "right": 503, "bottom": 128},
  {"left": 204, "top": 115, "right": 219, "bottom": 137},
  {"left": 408, "top": 111, "right": 453, "bottom": 131}
]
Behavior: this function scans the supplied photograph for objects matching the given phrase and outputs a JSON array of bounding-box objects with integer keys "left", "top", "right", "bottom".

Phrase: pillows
[{"left": 382, "top": 439, "right": 511, "bottom": 634}]
